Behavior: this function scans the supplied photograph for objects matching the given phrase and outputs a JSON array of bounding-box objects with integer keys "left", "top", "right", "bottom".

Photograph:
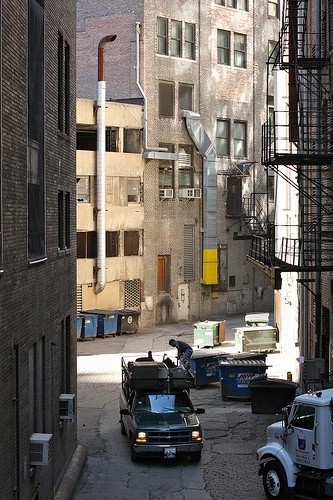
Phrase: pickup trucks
[{"left": 118, "top": 354, "right": 206, "bottom": 463}]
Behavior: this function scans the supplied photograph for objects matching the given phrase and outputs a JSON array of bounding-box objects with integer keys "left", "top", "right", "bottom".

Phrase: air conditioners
[
  {"left": 179, "top": 153, "right": 192, "bottom": 169},
  {"left": 58, "top": 393, "right": 77, "bottom": 420},
  {"left": 179, "top": 188, "right": 201, "bottom": 202},
  {"left": 29, "top": 433, "right": 53, "bottom": 466},
  {"left": 300, "top": 358, "right": 324, "bottom": 385},
  {"left": 159, "top": 188, "right": 173, "bottom": 201}
]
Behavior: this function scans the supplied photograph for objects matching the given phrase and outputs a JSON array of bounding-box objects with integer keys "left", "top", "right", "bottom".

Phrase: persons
[{"left": 169, "top": 339, "right": 193, "bottom": 364}]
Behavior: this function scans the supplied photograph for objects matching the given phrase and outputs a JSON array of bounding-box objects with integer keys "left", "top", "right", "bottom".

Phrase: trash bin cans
[
  {"left": 232, "top": 325, "right": 277, "bottom": 353},
  {"left": 217, "top": 360, "right": 268, "bottom": 400},
  {"left": 190, "top": 349, "right": 228, "bottom": 390},
  {"left": 192, "top": 319, "right": 226, "bottom": 349},
  {"left": 250, "top": 373, "right": 300, "bottom": 414},
  {"left": 245, "top": 312, "right": 269, "bottom": 326},
  {"left": 78, "top": 314, "right": 101, "bottom": 343},
  {"left": 213, "top": 352, "right": 267, "bottom": 363},
  {"left": 76, "top": 317, "right": 84, "bottom": 340},
  {"left": 81, "top": 309, "right": 118, "bottom": 340},
  {"left": 115, "top": 309, "right": 141, "bottom": 336}
]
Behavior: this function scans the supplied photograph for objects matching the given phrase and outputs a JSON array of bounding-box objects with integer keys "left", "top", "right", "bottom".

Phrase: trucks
[{"left": 255, "top": 389, "right": 333, "bottom": 499}]
